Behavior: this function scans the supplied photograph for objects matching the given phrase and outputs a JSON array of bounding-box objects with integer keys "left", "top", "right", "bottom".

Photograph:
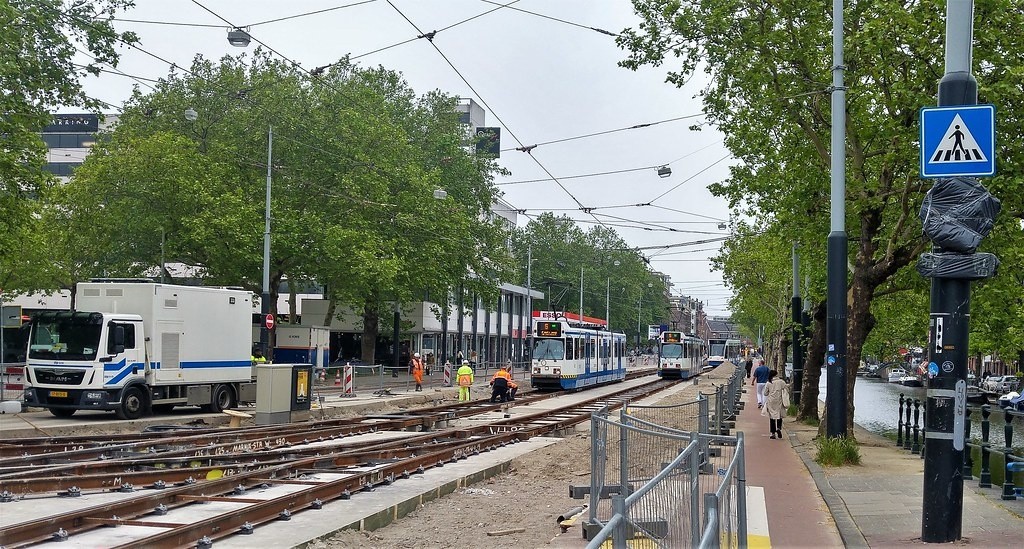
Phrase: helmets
[
  {"left": 463, "top": 359, "right": 469, "bottom": 363},
  {"left": 414, "top": 353, "right": 420, "bottom": 357}
]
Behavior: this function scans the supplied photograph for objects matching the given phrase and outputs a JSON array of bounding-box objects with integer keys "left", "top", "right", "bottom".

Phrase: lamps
[
  {"left": 717, "top": 222, "right": 726, "bottom": 231},
  {"left": 657, "top": 165, "right": 673, "bottom": 179},
  {"left": 184, "top": 107, "right": 199, "bottom": 122},
  {"left": 226, "top": 27, "right": 252, "bottom": 49},
  {"left": 432, "top": 188, "right": 448, "bottom": 201},
  {"left": 613, "top": 260, "right": 621, "bottom": 266},
  {"left": 647, "top": 282, "right": 654, "bottom": 288}
]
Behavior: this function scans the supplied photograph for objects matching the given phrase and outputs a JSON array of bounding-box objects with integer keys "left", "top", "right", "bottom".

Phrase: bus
[
  {"left": 655, "top": 331, "right": 705, "bottom": 379},
  {"left": 707, "top": 337, "right": 742, "bottom": 368},
  {"left": 525, "top": 318, "right": 627, "bottom": 390}
]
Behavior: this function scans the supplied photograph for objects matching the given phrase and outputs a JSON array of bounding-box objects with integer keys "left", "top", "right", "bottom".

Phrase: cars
[
  {"left": 967, "top": 372, "right": 1024, "bottom": 409},
  {"left": 888, "top": 368, "right": 924, "bottom": 388}
]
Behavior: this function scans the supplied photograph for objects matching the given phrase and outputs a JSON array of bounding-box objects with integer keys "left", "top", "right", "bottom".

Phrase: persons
[
  {"left": 457, "top": 349, "right": 464, "bottom": 368},
  {"left": 751, "top": 360, "right": 771, "bottom": 408},
  {"left": 507, "top": 382, "right": 518, "bottom": 400},
  {"left": 921, "top": 358, "right": 928, "bottom": 369},
  {"left": 469, "top": 351, "right": 477, "bottom": 375},
  {"left": 628, "top": 347, "right": 657, "bottom": 367},
  {"left": 984, "top": 369, "right": 991, "bottom": 380},
  {"left": 410, "top": 353, "right": 424, "bottom": 392},
  {"left": 745, "top": 359, "right": 753, "bottom": 378},
  {"left": 489, "top": 367, "right": 511, "bottom": 403},
  {"left": 251, "top": 347, "right": 267, "bottom": 361},
  {"left": 456, "top": 359, "right": 475, "bottom": 402},
  {"left": 761, "top": 370, "right": 791, "bottom": 439},
  {"left": 426, "top": 352, "right": 435, "bottom": 376}
]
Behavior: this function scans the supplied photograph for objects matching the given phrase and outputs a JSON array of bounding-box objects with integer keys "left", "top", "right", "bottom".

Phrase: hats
[{"left": 253, "top": 345, "right": 263, "bottom": 353}]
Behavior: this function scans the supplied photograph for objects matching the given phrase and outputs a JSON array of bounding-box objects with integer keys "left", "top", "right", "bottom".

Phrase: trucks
[{"left": 24, "top": 277, "right": 253, "bottom": 421}]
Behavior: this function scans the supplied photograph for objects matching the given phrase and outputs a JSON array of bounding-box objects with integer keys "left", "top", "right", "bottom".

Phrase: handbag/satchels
[{"left": 782, "top": 386, "right": 791, "bottom": 409}]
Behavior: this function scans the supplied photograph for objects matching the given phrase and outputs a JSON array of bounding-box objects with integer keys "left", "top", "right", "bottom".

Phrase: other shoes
[
  {"left": 770, "top": 435, "right": 775, "bottom": 439},
  {"left": 416, "top": 384, "right": 422, "bottom": 391},
  {"left": 776, "top": 429, "right": 782, "bottom": 438},
  {"left": 758, "top": 403, "right": 762, "bottom": 408}
]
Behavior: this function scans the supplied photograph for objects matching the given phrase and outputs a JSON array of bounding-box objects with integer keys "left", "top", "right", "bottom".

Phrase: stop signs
[{"left": 266, "top": 314, "right": 274, "bottom": 330}]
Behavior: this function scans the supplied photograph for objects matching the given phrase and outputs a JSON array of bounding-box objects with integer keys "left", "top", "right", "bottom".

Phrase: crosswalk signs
[{"left": 920, "top": 105, "right": 995, "bottom": 178}]
